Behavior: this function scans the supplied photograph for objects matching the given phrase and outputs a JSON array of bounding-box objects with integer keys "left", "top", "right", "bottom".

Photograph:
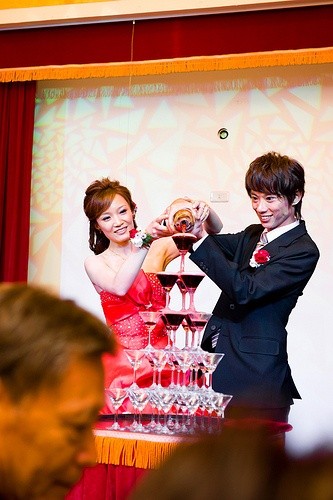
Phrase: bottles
[{"left": 166, "top": 197, "right": 197, "bottom": 234}]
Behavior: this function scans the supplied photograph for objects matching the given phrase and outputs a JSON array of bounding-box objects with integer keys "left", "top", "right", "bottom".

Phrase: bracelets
[{"left": 128, "top": 228, "right": 152, "bottom": 250}]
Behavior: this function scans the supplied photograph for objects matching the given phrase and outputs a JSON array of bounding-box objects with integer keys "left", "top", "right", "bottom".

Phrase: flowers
[
  {"left": 129, "top": 229, "right": 151, "bottom": 250},
  {"left": 249, "top": 250, "right": 271, "bottom": 268}
]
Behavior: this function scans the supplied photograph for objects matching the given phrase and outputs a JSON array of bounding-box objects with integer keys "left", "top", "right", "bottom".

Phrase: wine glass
[{"left": 102, "top": 233, "right": 233, "bottom": 437}]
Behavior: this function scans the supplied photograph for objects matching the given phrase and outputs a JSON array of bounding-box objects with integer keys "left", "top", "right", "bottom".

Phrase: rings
[{"left": 151, "top": 228, "right": 157, "bottom": 234}]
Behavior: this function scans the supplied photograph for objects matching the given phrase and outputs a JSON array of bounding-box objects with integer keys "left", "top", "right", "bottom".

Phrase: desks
[{"left": 65, "top": 422, "right": 292, "bottom": 500}]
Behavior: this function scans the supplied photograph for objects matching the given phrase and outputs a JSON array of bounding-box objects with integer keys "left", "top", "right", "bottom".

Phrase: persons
[
  {"left": 164, "top": 151, "right": 321, "bottom": 423},
  {"left": 82, "top": 177, "right": 227, "bottom": 415},
  {"left": 0, "top": 281, "right": 115, "bottom": 500}
]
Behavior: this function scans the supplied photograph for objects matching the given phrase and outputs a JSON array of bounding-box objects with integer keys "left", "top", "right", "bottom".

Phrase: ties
[{"left": 210, "top": 240, "right": 265, "bottom": 348}]
